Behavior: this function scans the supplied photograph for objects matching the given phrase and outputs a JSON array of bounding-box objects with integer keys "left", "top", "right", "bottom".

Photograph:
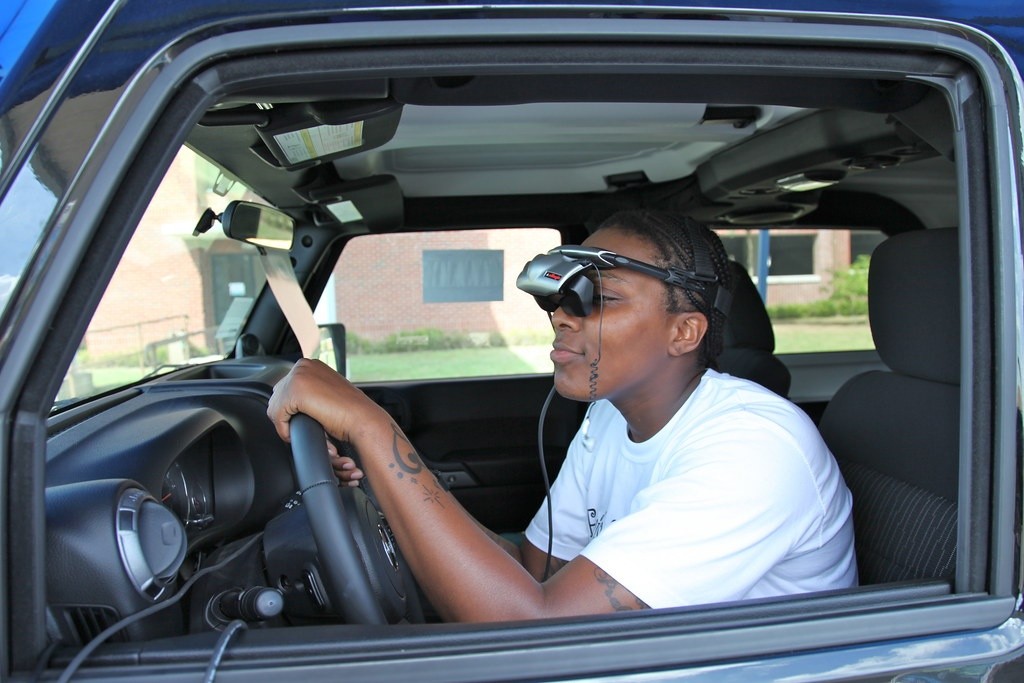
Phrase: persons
[{"left": 267, "top": 209, "right": 858, "bottom": 622}]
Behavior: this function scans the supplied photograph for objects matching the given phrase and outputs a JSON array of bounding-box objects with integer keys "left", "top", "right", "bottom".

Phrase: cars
[{"left": 0, "top": 0, "right": 1024, "bottom": 683}]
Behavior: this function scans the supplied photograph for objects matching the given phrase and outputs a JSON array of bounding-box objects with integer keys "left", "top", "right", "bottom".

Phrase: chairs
[
  {"left": 814, "top": 226, "right": 962, "bottom": 587},
  {"left": 711, "top": 259, "right": 793, "bottom": 403}
]
